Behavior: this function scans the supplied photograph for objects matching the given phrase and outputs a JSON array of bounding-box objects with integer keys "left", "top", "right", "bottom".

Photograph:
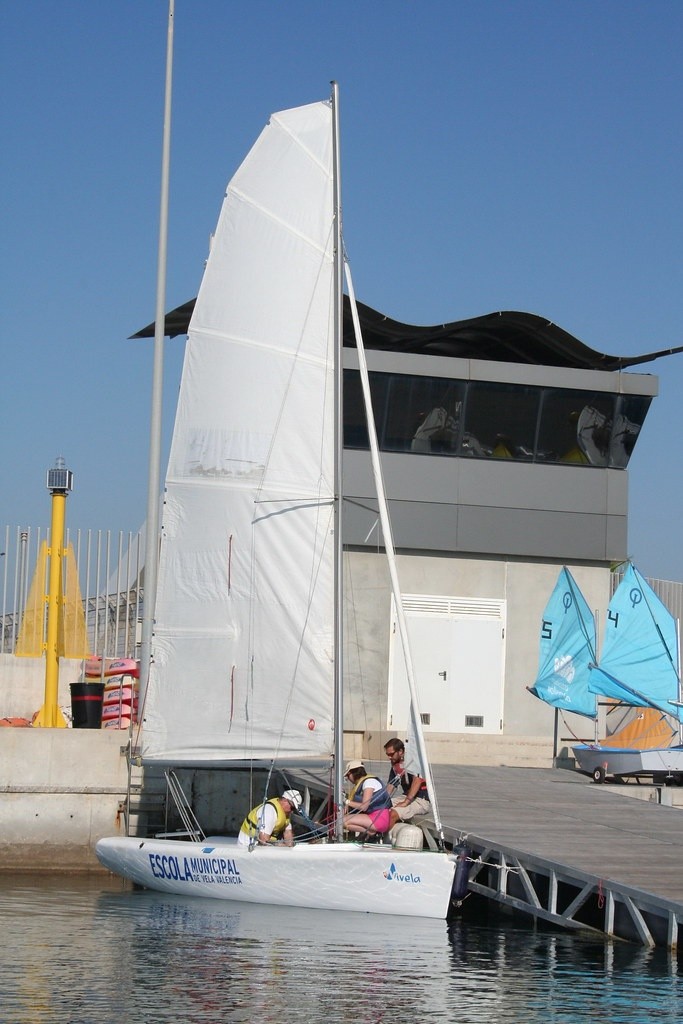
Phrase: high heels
[{"left": 359, "top": 831, "right": 380, "bottom": 844}]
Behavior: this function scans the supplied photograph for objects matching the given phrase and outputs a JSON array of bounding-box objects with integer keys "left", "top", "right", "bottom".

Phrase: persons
[
  {"left": 343, "top": 760, "right": 393, "bottom": 843},
  {"left": 237, "top": 790, "right": 303, "bottom": 847},
  {"left": 384, "top": 738, "right": 431, "bottom": 826}
]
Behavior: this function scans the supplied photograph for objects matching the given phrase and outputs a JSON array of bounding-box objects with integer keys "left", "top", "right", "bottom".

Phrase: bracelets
[
  {"left": 407, "top": 797, "right": 411, "bottom": 801},
  {"left": 345, "top": 798, "right": 350, "bottom": 805}
]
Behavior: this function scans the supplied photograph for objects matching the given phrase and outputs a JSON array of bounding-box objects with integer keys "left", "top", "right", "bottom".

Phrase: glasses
[
  {"left": 346, "top": 772, "right": 351, "bottom": 777},
  {"left": 287, "top": 800, "right": 296, "bottom": 812},
  {"left": 385, "top": 751, "right": 397, "bottom": 757}
]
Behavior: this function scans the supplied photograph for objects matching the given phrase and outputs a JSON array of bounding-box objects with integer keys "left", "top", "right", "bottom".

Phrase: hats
[
  {"left": 343, "top": 760, "right": 365, "bottom": 777},
  {"left": 282, "top": 790, "right": 302, "bottom": 810}
]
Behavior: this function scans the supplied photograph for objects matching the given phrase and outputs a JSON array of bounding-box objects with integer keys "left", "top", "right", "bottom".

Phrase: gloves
[{"left": 342, "top": 792, "right": 347, "bottom": 802}]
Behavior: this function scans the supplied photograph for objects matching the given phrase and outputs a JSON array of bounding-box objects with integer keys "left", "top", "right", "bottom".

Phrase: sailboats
[
  {"left": 96, "top": 80, "right": 460, "bottom": 927},
  {"left": 526, "top": 558, "right": 682, "bottom": 787}
]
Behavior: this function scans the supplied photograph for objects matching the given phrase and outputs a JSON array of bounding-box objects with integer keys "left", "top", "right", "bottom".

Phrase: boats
[{"left": 77, "top": 657, "right": 140, "bottom": 730}]
